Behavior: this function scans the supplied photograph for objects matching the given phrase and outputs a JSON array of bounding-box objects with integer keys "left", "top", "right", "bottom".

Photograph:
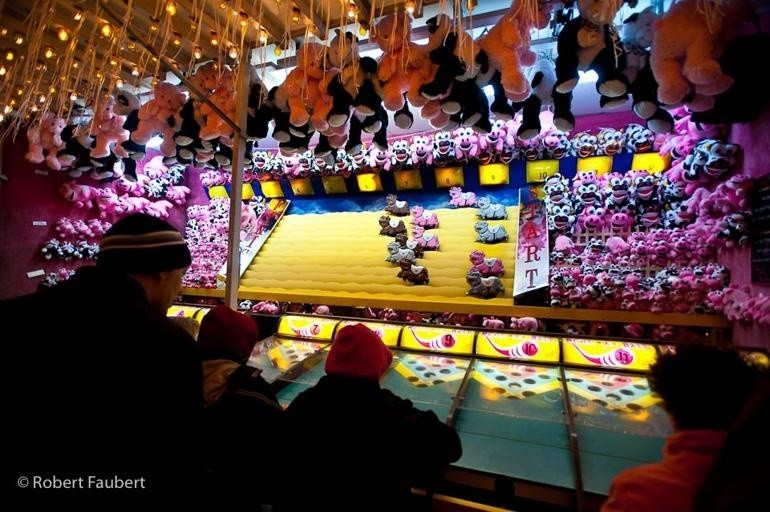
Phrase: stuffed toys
[
  {"left": 24, "top": 2, "right": 769, "bottom": 342},
  {"left": 25, "top": 0, "right": 770, "bottom": 341}
]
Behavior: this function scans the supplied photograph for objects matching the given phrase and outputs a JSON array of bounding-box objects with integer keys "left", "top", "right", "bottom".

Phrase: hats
[
  {"left": 324, "top": 322, "right": 394, "bottom": 378},
  {"left": 96, "top": 211, "right": 192, "bottom": 278},
  {"left": 196, "top": 304, "right": 259, "bottom": 364}
]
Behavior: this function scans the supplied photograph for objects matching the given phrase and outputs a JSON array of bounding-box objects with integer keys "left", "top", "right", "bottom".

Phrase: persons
[
  {"left": 0, "top": 211, "right": 205, "bottom": 511},
  {"left": 195, "top": 301, "right": 284, "bottom": 512},
  {"left": 595, "top": 342, "right": 770, "bottom": 511},
  {"left": 264, "top": 322, "right": 464, "bottom": 512}
]
